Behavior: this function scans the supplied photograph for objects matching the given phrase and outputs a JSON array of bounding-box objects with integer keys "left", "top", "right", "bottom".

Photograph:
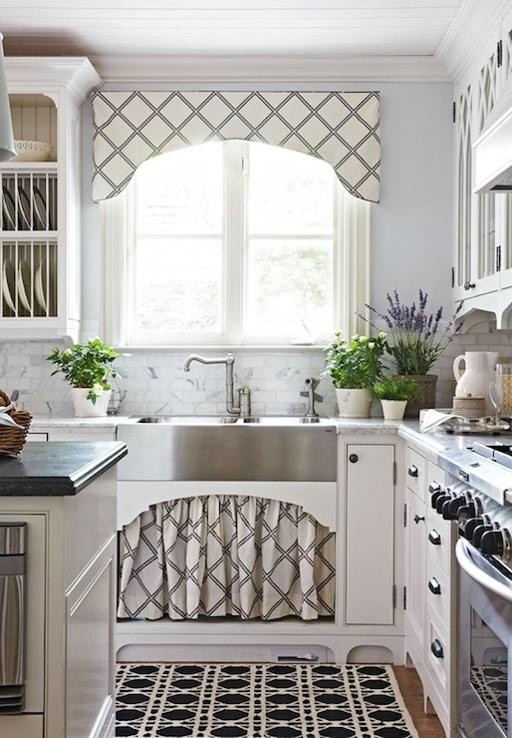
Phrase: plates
[
  {"left": 440, "top": 421, "right": 508, "bottom": 436},
  {"left": 2, "top": 171, "right": 57, "bottom": 318}
]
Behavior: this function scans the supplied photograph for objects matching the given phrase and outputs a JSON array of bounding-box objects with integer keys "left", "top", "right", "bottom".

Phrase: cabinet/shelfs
[
  {"left": 0, "top": 55, "right": 104, "bottom": 347},
  {"left": 405, "top": 446, "right": 452, "bottom": 712},
  {"left": 346, "top": 444, "right": 395, "bottom": 626}
]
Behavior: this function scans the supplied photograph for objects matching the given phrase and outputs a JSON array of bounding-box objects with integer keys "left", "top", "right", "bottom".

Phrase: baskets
[{"left": 0, "top": 385, "right": 35, "bottom": 464}]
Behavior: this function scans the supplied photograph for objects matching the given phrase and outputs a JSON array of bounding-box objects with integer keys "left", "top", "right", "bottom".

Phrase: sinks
[
  {"left": 138, "top": 416, "right": 239, "bottom": 425},
  {"left": 239, "top": 415, "right": 320, "bottom": 425}
]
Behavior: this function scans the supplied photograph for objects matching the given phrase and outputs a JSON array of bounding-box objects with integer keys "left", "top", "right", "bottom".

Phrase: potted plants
[
  {"left": 46, "top": 338, "right": 119, "bottom": 417},
  {"left": 371, "top": 375, "right": 420, "bottom": 420}
]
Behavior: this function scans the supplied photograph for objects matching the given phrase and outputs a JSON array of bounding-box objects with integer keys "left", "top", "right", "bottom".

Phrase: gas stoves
[{"left": 427, "top": 442, "right": 512, "bottom": 577}]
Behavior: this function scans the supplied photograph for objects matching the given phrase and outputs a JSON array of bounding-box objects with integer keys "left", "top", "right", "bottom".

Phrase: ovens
[{"left": 450, "top": 534, "right": 512, "bottom": 738}]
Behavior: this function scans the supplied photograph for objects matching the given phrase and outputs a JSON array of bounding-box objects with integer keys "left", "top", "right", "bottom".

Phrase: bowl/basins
[{"left": 10, "top": 140, "right": 53, "bottom": 160}]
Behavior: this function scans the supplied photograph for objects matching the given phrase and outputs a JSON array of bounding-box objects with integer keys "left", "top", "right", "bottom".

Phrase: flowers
[
  {"left": 354, "top": 289, "right": 466, "bottom": 375},
  {"left": 323, "top": 331, "right": 387, "bottom": 389}
]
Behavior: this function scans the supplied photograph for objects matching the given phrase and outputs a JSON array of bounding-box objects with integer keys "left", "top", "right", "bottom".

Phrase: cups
[
  {"left": 452, "top": 398, "right": 485, "bottom": 419},
  {"left": 487, "top": 362, "right": 512, "bottom": 421},
  {"left": 450, "top": 350, "right": 500, "bottom": 417}
]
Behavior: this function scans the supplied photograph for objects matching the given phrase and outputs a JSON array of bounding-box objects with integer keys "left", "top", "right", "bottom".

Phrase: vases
[
  {"left": 392, "top": 372, "right": 438, "bottom": 418},
  {"left": 335, "top": 387, "right": 372, "bottom": 417}
]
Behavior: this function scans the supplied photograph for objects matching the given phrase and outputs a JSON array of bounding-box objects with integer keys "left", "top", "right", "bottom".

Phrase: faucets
[
  {"left": 299, "top": 377, "right": 323, "bottom": 416},
  {"left": 181, "top": 352, "right": 250, "bottom": 416}
]
